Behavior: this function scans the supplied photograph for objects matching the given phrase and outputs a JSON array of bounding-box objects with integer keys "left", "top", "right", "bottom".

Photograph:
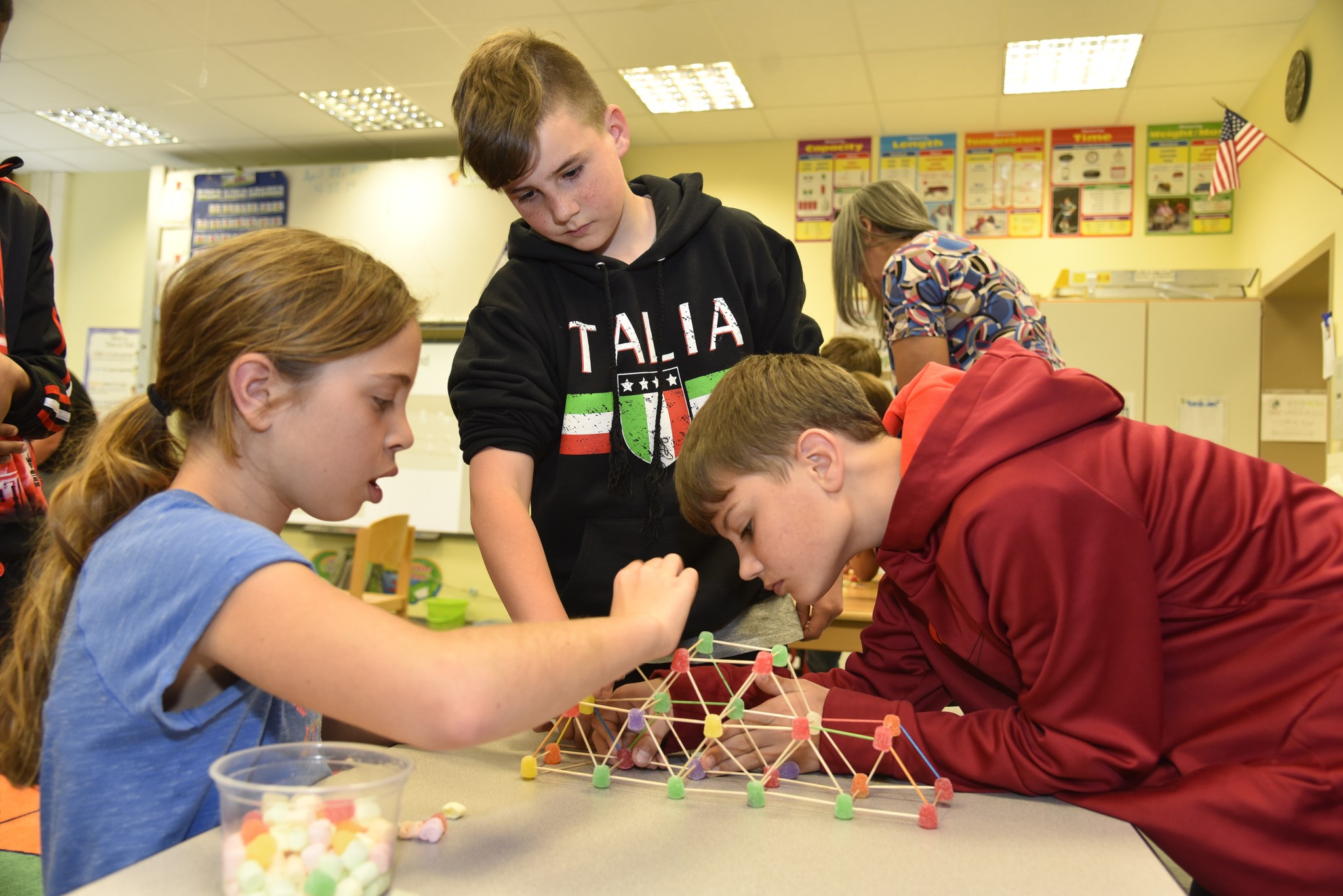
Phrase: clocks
[{"left": 1285, "top": 50, "right": 1306, "bottom": 122}]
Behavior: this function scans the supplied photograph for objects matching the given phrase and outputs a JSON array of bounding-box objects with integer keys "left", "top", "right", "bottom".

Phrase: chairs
[{"left": 343, "top": 515, "right": 416, "bottom": 619}]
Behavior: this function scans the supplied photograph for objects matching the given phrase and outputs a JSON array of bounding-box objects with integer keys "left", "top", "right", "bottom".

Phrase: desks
[{"left": 59, "top": 728, "right": 1191, "bottom": 896}]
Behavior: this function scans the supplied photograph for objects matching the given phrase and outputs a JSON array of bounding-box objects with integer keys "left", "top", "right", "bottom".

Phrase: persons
[
  {"left": 977, "top": 216, "right": 995, "bottom": 234},
  {"left": 1153, "top": 199, "right": 1188, "bottom": 231},
  {"left": 929, "top": 204, "right": 952, "bottom": 232},
  {"left": 587, "top": 352, "right": 1342, "bottom": 889},
  {"left": 821, "top": 335, "right": 907, "bottom": 581},
  {"left": 1057, "top": 196, "right": 1077, "bottom": 234},
  {"left": 450, "top": 32, "right": 826, "bottom": 756},
  {"left": 0, "top": 227, "right": 698, "bottom": 896},
  {"left": 830, "top": 178, "right": 1066, "bottom": 390},
  {"left": 0, "top": 0, "right": 73, "bottom": 654}
]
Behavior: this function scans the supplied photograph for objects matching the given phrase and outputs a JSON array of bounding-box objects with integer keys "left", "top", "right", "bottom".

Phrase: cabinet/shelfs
[{"left": 1041, "top": 302, "right": 1262, "bottom": 458}]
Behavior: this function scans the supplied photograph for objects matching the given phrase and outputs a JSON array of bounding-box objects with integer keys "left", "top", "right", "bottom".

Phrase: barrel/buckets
[{"left": 424, "top": 598, "right": 470, "bottom": 629}]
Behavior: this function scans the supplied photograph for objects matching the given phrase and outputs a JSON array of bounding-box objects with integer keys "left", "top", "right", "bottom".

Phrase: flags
[{"left": 1209, "top": 108, "right": 1267, "bottom": 199}]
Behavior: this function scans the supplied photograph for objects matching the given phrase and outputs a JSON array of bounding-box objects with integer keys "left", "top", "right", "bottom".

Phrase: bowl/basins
[{"left": 206, "top": 740, "right": 413, "bottom": 896}]
